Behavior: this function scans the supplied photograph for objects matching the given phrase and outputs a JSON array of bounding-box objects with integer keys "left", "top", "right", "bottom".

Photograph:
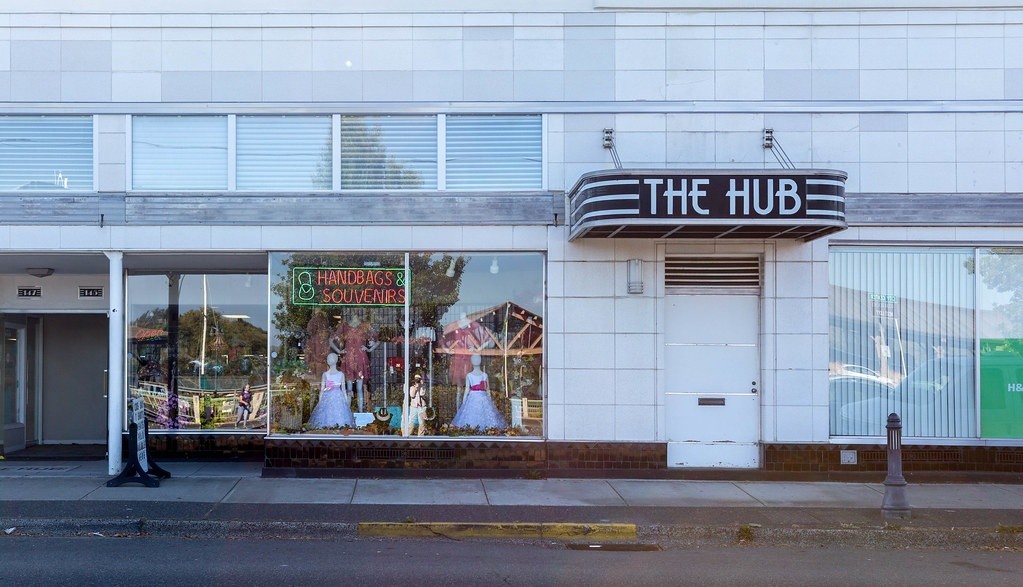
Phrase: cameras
[{"left": 414, "top": 378, "right": 420, "bottom": 383}]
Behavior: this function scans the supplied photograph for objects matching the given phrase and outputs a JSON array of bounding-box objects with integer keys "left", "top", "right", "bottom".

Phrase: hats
[{"left": 415, "top": 374, "right": 422, "bottom": 379}]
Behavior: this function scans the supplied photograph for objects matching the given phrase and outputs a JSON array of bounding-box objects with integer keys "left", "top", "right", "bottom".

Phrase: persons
[
  {"left": 406, "top": 374, "right": 428, "bottom": 437},
  {"left": 440, "top": 311, "right": 494, "bottom": 415},
  {"left": 234, "top": 383, "right": 253, "bottom": 429},
  {"left": 450, "top": 353, "right": 509, "bottom": 429},
  {"left": 306, "top": 352, "right": 358, "bottom": 429},
  {"left": 329, "top": 309, "right": 381, "bottom": 413},
  {"left": 304, "top": 301, "right": 331, "bottom": 417}
]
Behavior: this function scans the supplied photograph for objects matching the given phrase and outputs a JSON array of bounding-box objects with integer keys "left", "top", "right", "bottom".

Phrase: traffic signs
[
  {"left": 872, "top": 306, "right": 899, "bottom": 320},
  {"left": 868, "top": 293, "right": 897, "bottom": 303}
]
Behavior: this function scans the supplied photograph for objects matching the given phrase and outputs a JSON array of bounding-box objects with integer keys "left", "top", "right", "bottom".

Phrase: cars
[
  {"left": 830, "top": 355, "right": 1022, "bottom": 437},
  {"left": 182, "top": 353, "right": 267, "bottom": 375}
]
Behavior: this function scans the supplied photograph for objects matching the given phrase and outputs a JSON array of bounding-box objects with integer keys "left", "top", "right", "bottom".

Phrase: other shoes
[
  {"left": 243, "top": 425, "right": 247, "bottom": 429},
  {"left": 235, "top": 422, "right": 238, "bottom": 427}
]
